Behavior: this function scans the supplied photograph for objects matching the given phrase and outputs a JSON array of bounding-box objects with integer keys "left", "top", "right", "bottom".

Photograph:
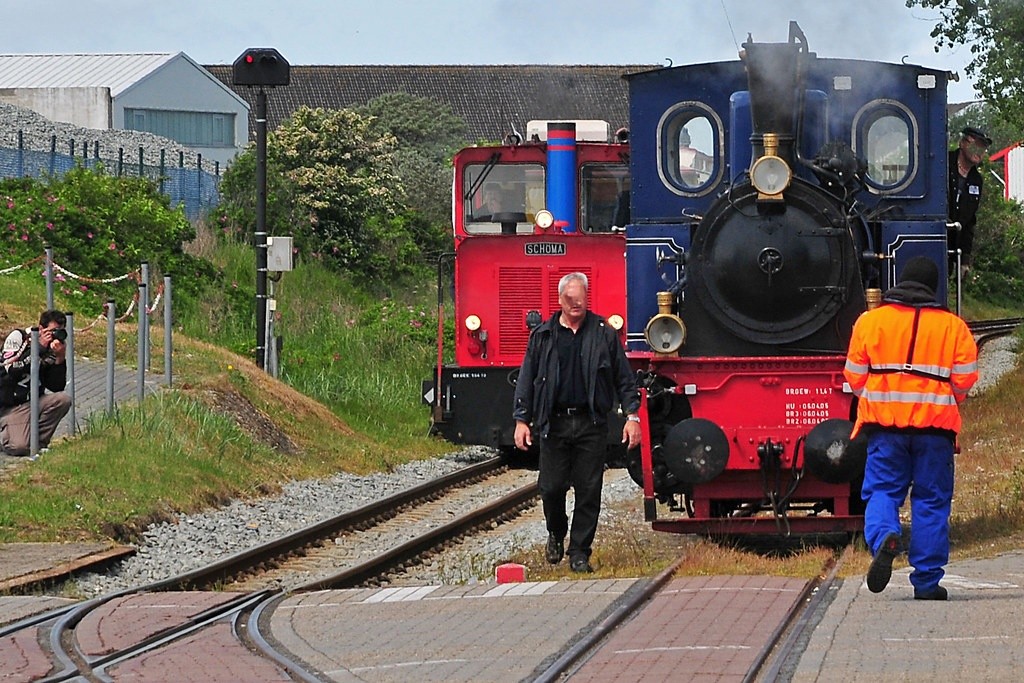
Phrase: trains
[
  {"left": 617, "top": 18, "right": 965, "bottom": 537},
  {"left": 427, "top": 120, "right": 712, "bottom": 453}
]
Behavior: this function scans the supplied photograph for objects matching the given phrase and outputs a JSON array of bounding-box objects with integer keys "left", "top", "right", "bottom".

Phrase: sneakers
[
  {"left": 545, "top": 528, "right": 568, "bottom": 564},
  {"left": 570, "top": 552, "right": 593, "bottom": 573}
]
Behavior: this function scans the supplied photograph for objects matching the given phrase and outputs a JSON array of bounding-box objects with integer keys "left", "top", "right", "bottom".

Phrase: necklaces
[{"left": 958, "top": 161, "right": 968, "bottom": 173}]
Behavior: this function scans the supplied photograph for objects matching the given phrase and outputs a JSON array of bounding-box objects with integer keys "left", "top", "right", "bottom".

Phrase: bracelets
[{"left": 628, "top": 417, "right": 640, "bottom": 422}]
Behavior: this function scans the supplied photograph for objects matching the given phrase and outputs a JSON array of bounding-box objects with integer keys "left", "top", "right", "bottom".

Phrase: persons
[
  {"left": 845, "top": 255, "right": 978, "bottom": 598},
  {"left": 0, "top": 309, "right": 71, "bottom": 456},
  {"left": 948, "top": 126, "right": 992, "bottom": 277},
  {"left": 473, "top": 182, "right": 526, "bottom": 223},
  {"left": 514, "top": 273, "right": 643, "bottom": 574}
]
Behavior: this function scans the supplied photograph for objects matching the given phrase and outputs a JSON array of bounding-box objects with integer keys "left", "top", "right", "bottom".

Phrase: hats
[
  {"left": 900, "top": 257, "right": 938, "bottom": 294},
  {"left": 963, "top": 127, "right": 991, "bottom": 146}
]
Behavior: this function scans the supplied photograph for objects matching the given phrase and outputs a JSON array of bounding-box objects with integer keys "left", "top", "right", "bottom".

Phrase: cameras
[{"left": 47, "top": 327, "right": 68, "bottom": 349}]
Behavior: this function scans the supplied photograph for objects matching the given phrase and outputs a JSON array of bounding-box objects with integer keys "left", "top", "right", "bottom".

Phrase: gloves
[{"left": 957, "top": 264, "right": 970, "bottom": 282}]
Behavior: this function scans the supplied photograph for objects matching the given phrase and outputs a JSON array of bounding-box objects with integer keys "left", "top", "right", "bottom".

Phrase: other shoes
[
  {"left": 914, "top": 586, "right": 948, "bottom": 599},
  {"left": 866, "top": 534, "right": 900, "bottom": 593},
  {"left": 39, "top": 447, "right": 49, "bottom": 455}
]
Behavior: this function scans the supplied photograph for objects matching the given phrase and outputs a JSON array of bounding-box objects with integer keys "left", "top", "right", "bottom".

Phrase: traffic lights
[{"left": 231, "top": 47, "right": 293, "bottom": 87}]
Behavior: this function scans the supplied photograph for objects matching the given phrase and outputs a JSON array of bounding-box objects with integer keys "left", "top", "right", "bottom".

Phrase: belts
[{"left": 552, "top": 407, "right": 589, "bottom": 416}]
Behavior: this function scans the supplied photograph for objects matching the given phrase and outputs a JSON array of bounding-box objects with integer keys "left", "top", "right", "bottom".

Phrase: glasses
[{"left": 963, "top": 136, "right": 988, "bottom": 155}]
